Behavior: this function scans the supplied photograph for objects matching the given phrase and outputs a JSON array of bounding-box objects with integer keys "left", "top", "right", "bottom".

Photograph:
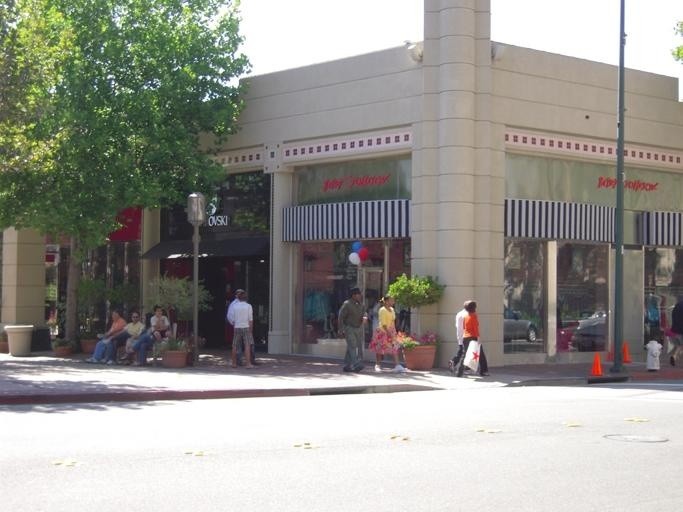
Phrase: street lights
[{"left": 184, "top": 187, "right": 209, "bottom": 370}]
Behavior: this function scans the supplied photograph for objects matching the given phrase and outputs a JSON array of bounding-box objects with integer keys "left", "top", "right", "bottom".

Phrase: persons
[
  {"left": 232, "top": 292, "right": 257, "bottom": 369},
  {"left": 448, "top": 300, "right": 468, "bottom": 373},
  {"left": 456, "top": 301, "right": 490, "bottom": 376},
  {"left": 338, "top": 287, "right": 407, "bottom": 373},
  {"left": 671, "top": 300, "right": 683, "bottom": 365},
  {"left": 84, "top": 306, "right": 171, "bottom": 366},
  {"left": 227, "top": 289, "right": 258, "bottom": 366}
]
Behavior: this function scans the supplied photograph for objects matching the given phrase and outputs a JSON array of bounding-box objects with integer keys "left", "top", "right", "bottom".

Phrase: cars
[{"left": 501, "top": 295, "right": 607, "bottom": 354}]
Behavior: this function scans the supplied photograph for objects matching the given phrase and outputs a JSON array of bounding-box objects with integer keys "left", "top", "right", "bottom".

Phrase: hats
[{"left": 349, "top": 288, "right": 361, "bottom": 296}]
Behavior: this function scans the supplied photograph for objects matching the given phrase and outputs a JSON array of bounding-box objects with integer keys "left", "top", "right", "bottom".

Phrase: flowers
[{"left": 411, "top": 329, "right": 439, "bottom": 346}]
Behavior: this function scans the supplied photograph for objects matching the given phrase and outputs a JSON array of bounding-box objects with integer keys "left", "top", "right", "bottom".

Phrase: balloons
[
  {"left": 357, "top": 248, "right": 368, "bottom": 261},
  {"left": 348, "top": 252, "right": 361, "bottom": 265},
  {"left": 352, "top": 242, "right": 361, "bottom": 254}
]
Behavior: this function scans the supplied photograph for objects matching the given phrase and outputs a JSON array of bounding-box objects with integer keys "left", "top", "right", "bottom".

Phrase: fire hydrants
[{"left": 643, "top": 339, "right": 664, "bottom": 372}]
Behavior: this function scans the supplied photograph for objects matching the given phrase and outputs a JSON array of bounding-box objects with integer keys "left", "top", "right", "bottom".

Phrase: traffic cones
[
  {"left": 622, "top": 342, "right": 632, "bottom": 363},
  {"left": 590, "top": 351, "right": 603, "bottom": 376}
]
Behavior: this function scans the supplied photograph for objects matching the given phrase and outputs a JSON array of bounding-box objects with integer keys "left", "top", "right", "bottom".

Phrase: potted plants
[
  {"left": 161, "top": 335, "right": 188, "bottom": 367},
  {"left": 78, "top": 280, "right": 105, "bottom": 353}
]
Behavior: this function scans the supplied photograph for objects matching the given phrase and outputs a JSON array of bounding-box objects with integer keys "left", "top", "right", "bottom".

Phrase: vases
[{"left": 402, "top": 343, "right": 436, "bottom": 368}]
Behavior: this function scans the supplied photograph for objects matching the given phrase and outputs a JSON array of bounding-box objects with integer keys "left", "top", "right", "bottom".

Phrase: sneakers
[
  {"left": 354, "top": 364, "right": 364, "bottom": 372},
  {"left": 86, "top": 354, "right": 141, "bottom": 365},
  {"left": 395, "top": 365, "right": 407, "bottom": 372},
  {"left": 375, "top": 365, "right": 382, "bottom": 371},
  {"left": 448, "top": 360, "right": 454, "bottom": 372},
  {"left": 344, "top": 366, "right": 355, "bottom": 372}
]
